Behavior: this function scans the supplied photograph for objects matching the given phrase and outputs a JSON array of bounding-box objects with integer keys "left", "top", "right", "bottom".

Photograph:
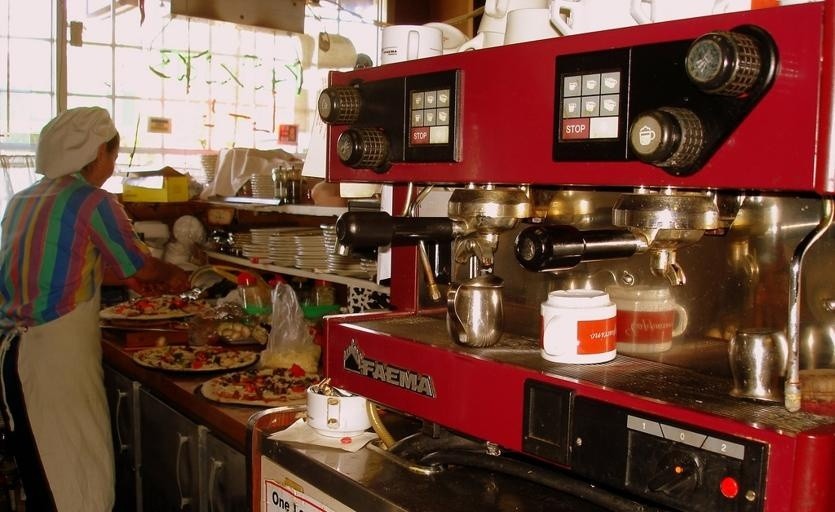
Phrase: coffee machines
[{"left": 317, "top": 1, "right": 830, "bottom": 511}]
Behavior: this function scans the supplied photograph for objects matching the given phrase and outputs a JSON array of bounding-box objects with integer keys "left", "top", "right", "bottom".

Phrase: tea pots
[{"left": 445, "top": 272, "right": 505, "bottom": 350}]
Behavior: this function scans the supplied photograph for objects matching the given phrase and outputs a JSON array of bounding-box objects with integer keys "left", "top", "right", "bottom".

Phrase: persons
[{"left": 1, "top": 105, "right": 194, "bottom": 511}]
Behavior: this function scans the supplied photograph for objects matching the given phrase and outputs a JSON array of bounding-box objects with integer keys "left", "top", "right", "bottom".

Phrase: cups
[
  {"left": 606, "top": 282, "right": 690, "bottom": 355},
  {"left": 459, "top": 0, "right": 752, "bottom": 58},
  {"left": 380, "top": 24, "right": 443, "bottom": 66},
  {"left": 537, "top": 287, "right": 618, "bottom": 364},
  {"left": 304, "top": 383, "right": 371, "bottom": 438}
]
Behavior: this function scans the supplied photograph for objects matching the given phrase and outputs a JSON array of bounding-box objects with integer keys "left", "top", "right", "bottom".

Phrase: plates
[
  {"left": 292, "top": 233, "right": 328, "bottom": 270},
  {"left": 249, "top": 173, "right": 271, "bottom": 200},
  {"left": 266, "top": 230, "right": 296, "bottom": 267},
  {"left": 232, "top": 225, "right": 267, "bottom": 262},
  {"left": 319, "top": 218, "right": 379, "bottom": 279},
  {"left": 201, "top": 153, "right": 218, "bottom": 186},
  {"left": 422, "top": 23, "right": 468, "bottom": 55}
]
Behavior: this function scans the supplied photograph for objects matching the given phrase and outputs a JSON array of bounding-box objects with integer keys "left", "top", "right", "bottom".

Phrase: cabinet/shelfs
[
  {"left": 191, "top": 194, "right": 392, "bottom": 297},
  {"left": 100, "top": 358, "right": 139, "bottom": 512},
  {"left": 138, "top": 388, "right": 254, "bottom": 512}
]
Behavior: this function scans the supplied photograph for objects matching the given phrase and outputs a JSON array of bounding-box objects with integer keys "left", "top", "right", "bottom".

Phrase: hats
[{"left": 35, "top": 106, "right": 118, "bottom": 180}]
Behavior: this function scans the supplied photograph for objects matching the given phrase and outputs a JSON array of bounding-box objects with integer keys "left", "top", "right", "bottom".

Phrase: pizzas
[
  {"left": 200, "top": 362, "right": 321, "bottom": 407},
  {"left": 98, "top": 293, "right": 217, "bottom": 320},
  {"left": 132, "top": 345, "right": 259, "bottom": 372}
]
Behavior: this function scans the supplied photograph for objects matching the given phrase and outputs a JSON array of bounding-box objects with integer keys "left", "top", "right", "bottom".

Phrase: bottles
[
  {"left": 285, "top": 164, "right": 303, "bottom": 205},
  {"left": 270, "top": 163, "right": 288, "bottom": 206}
]
